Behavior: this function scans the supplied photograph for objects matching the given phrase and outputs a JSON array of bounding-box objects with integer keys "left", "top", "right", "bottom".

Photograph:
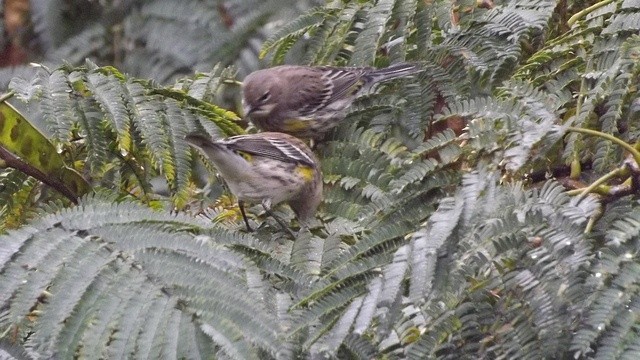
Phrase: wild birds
[
  {"left": 182, "top": 126, "right": 324, "bottom": 237},
  {"left": 239, "top": 63, "right": 377, "bottom": 150}
]
